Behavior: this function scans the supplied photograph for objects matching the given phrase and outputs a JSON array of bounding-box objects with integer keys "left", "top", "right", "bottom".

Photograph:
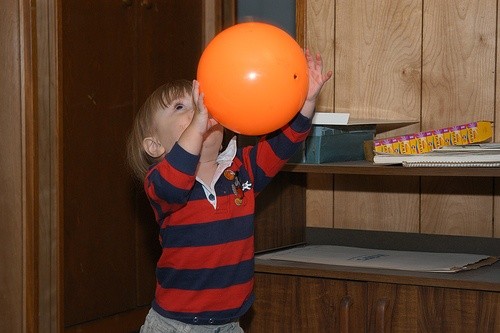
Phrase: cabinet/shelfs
[{"left": 214, "top": 0, "right": 500, "bottom": 333}]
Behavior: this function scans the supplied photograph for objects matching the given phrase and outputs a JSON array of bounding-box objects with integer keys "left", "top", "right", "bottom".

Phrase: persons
[{"left": 128, "top": 48, "right": 333, "bottom": 333}]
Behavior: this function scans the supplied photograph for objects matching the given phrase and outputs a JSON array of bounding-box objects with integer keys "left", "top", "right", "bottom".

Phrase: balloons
[{"left": 196, "top": 22, "right": 310, "bottom": 136}]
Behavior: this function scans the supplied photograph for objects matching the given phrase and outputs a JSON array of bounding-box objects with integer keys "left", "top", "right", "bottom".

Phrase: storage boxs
[
  {"left": 364, "top": 121, "right": 495, "bottom": 161},
  {"left": 286, "top": 126, "right": 377, "bottom": 164}
]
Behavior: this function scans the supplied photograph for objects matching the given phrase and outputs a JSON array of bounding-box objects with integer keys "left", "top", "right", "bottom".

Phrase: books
[{"left": 372, "top": 154, "right": 500, "bottom": 168}]
